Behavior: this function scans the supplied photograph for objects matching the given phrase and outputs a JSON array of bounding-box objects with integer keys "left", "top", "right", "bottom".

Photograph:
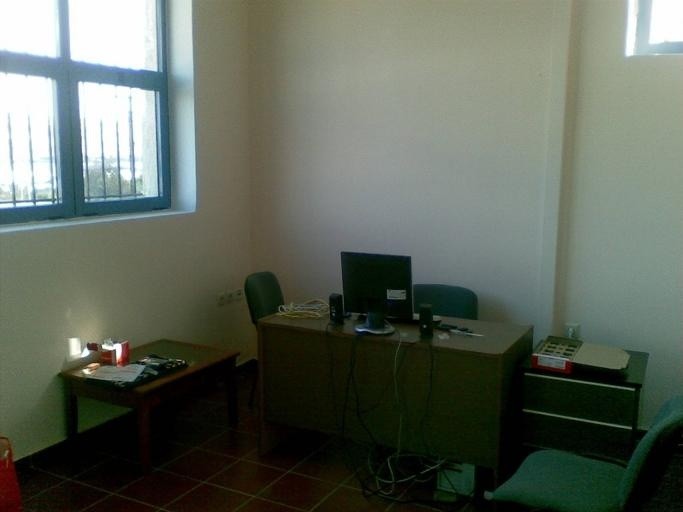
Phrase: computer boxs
[{"left": 437, "top": 456, "right": 475, "bottom": 496}]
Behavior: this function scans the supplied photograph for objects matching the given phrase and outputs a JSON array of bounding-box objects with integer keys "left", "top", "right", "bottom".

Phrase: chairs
[
  {"left": 246, "top": 272, "right": 285, "bottom": 326},
  {"left": 485, "top": 395, "right": 683, "bottom": 512},
  {"left": 408, "top": 283, "right": 479, "bottom": 321}
]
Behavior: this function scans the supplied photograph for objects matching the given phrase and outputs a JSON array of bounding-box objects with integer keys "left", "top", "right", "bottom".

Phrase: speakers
[
  {"left": 328, "top": 293, "right": 343, "bottom": 328},
  {"left": 419, "top": 304, "right": 434, "bottom": 338}
]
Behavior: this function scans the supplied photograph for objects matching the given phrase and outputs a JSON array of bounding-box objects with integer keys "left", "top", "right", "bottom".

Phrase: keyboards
[{"left": 358, "top": 313, "right": 442, "bottom": 324}]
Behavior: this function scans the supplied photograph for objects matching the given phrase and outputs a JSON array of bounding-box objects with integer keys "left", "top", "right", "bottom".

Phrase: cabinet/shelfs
[{"left": 520, "top": 349, "right": 649, "bottom": 463}]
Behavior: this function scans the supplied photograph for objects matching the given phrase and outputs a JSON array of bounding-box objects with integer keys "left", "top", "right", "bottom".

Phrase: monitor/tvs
[{"left": 340, "top": 251, "right": 414, "bottom": 335}]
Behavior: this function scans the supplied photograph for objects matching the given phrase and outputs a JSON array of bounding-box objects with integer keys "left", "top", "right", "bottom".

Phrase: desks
[
  {"left": 59, "top": 337, "right": 243, "bottom": 462},
  {"left": 257, "top": 311, "right": 533, "bottom": 484}
]
